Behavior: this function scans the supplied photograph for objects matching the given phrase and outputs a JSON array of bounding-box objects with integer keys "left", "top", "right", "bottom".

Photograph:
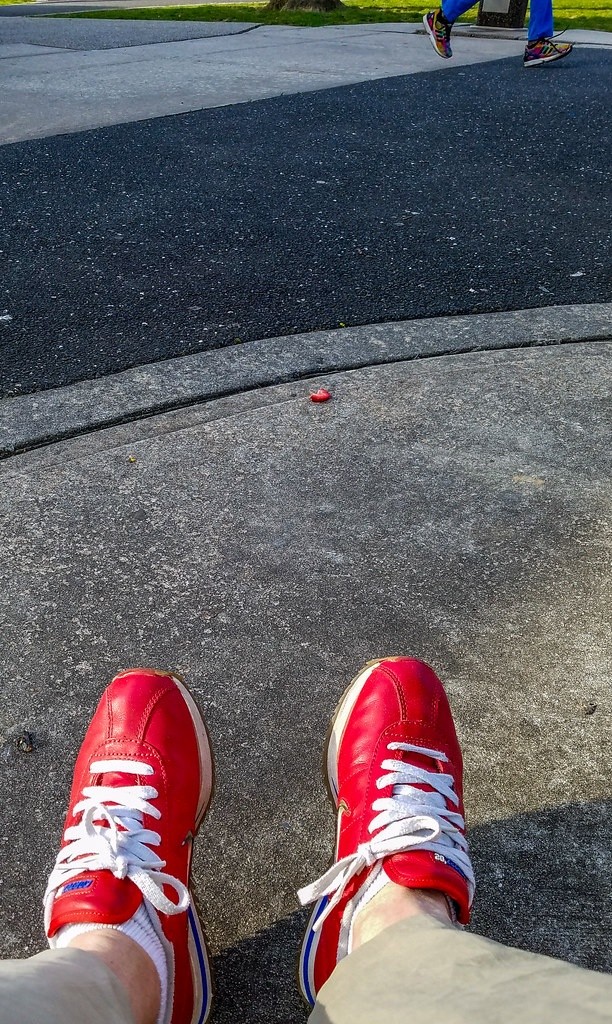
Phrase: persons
[
  {"left": 422, "top": 0, "right": 575, "bottom": 69},
  {"left": 0, "top": 653, "right": 612, "bottom": 1024}
]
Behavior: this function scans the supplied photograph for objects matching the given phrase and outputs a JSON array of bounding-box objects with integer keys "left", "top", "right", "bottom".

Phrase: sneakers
[
  {"left": 422, "top": 8, "right": 452, "bottom": 59},
  {"left": 295, "top": 655, "right": 476, "bottom": 1010},
  {"left": 523, "top": 25, "right": 573, "bottom": 68},
  {"left": 43, "top": 668, "right": 211, "bottom": 1024}
]
